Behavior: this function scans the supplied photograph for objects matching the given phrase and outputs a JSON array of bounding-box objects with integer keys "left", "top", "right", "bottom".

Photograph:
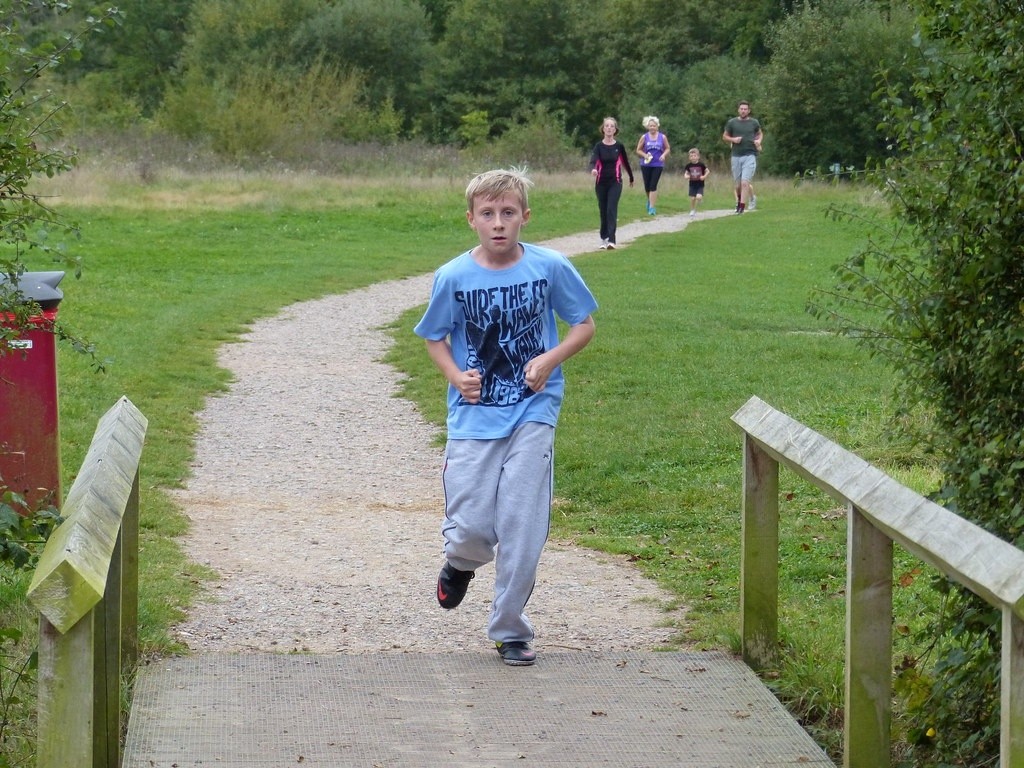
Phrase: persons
[
  {"left": 412, "top": 165, "right": 599, "bottom": 666},
  {"left": 685, "top": 148, "right": 710, "bottom": 220},
  {"left": 722, "top": 101, "right": 763, "bottom": 215},
  {"left": 731, "top": 140, "right": 762, "bottom": 209},
  {"left": 637, "top": 115, "right": 670, "bottom": 215},
  {"left": 588, "top": 117, "right": 633, "bottom": 250}
]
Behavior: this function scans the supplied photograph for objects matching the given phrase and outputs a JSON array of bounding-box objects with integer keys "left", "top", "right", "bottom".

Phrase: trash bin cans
[{"left": 1, "top": 269, "right": 65, "bottom": 519}]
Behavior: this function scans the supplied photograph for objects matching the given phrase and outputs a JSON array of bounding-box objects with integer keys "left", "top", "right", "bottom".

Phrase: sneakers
[
  {"left": 495, "top": 640, "right": 537, "bottom": 666},
  {"left": 646, "top": 200, "right": 655, "bottom": 215},
  {"left": 599, "top": 239, "right": 615, "bottom": 249},
  {"left": 437, "top": 562, "right": 474, "bottom": 610}
]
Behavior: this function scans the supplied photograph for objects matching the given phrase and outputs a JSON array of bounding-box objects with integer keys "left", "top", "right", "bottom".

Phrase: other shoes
[
  {"left": 690, "top": 209, "right": 696, "bottom": 216},
  {"left": 735, "top": 195, "right": 757, "bottom": 214}
]
